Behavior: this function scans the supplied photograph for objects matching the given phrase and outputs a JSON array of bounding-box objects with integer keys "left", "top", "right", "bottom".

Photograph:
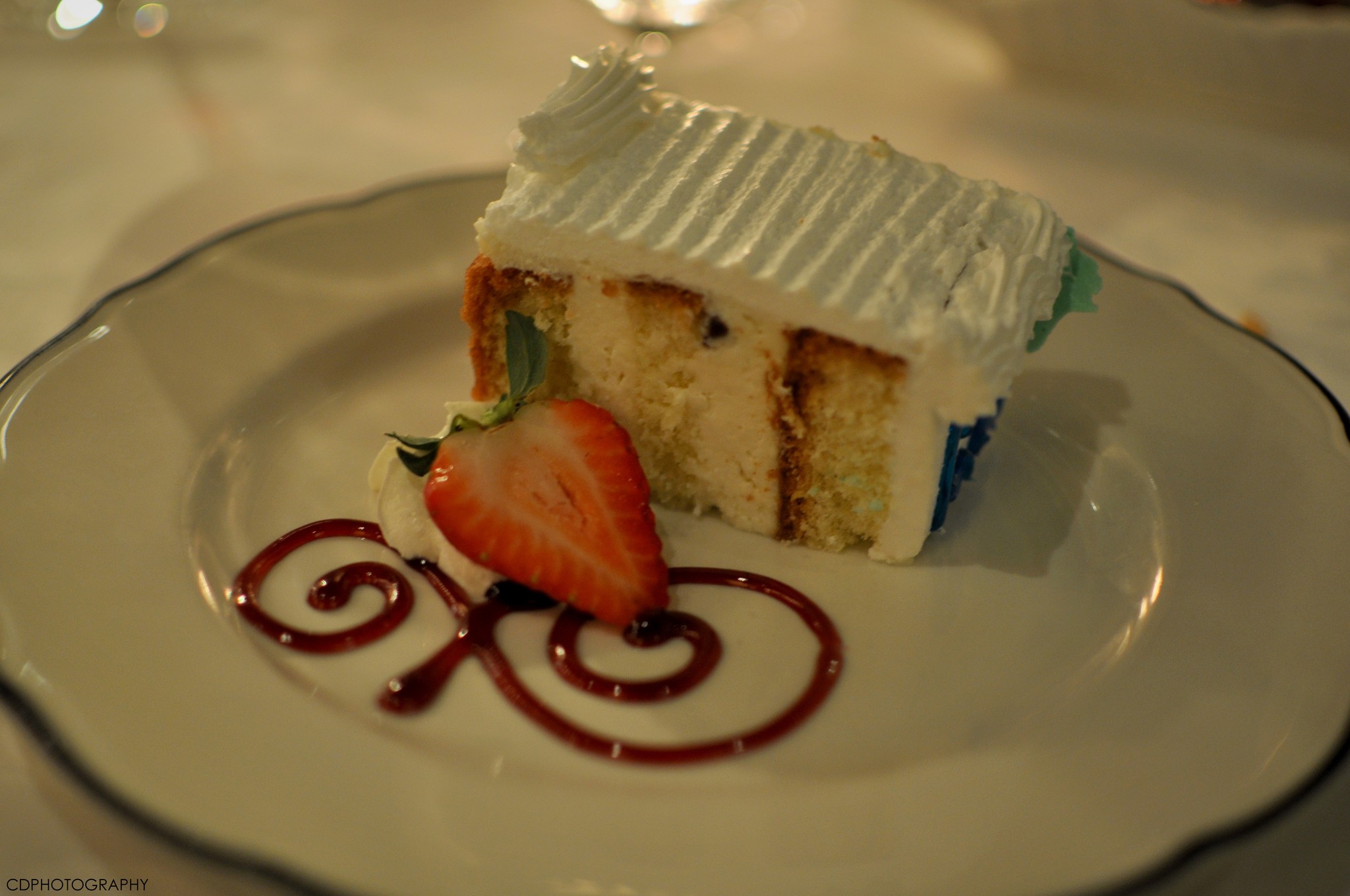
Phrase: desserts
[{"left": 464, "top": 43, "right": 1104, "bottom": 563}]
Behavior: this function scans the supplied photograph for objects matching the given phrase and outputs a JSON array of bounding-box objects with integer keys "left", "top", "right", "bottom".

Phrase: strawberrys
[{"left": 381, "top": 309, "right": 669, "bottom": 636}]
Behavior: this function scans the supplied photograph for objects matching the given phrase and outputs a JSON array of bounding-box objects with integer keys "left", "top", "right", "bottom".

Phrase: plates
[{"left": 0, "top": 160, "right": 1350, "bottom": 894}]
